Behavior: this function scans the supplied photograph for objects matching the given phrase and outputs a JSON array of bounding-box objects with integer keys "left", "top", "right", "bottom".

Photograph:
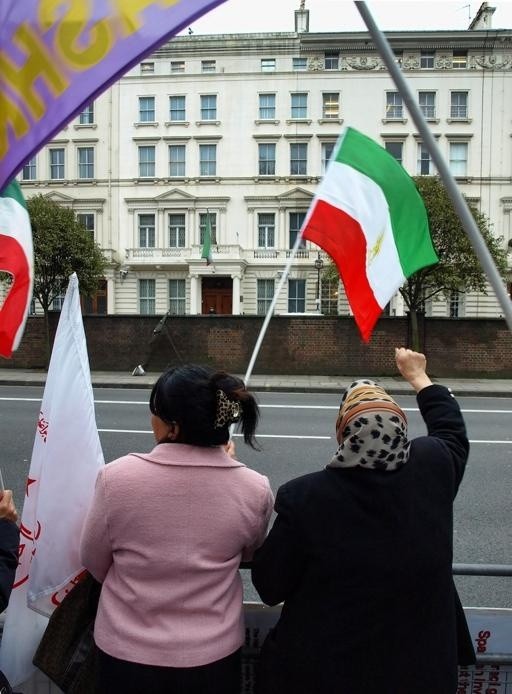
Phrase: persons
[
  {"left": 0, "top": 487, "right": 18, "bottom": 610},
  {"left": 30, "top": 362, "right": 274, "bottom": 692},
  {"left": 249, "top": 347, "right": 479, "bottom": 692}
]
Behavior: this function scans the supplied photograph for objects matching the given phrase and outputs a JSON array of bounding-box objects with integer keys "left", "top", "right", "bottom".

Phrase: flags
[
  {"left": 1, "top": 270, "right": 110, "bottom": 690},
  {"left": 0, "top": 180, "right": 34, "bottom": 359},
  {"left": 1, "top": 0, "right": 226, "bottom": 198},
  {"left": 201, "top": 210, "right": 212, "bottom": 265},
  {"left": 299, "top": 125, "right": 439, "bottom": 343}
]
